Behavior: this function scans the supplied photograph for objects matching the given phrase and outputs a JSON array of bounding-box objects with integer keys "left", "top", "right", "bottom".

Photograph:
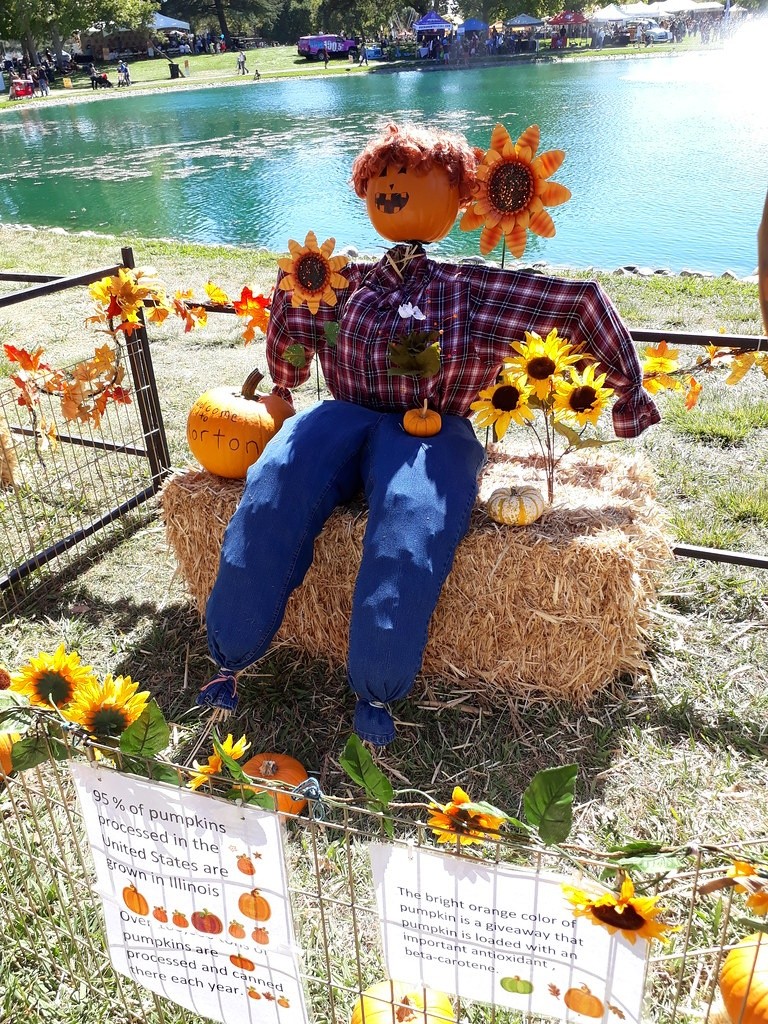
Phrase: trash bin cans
[{"left": 167, "top": 63, "right": 179, "bottom": 78}]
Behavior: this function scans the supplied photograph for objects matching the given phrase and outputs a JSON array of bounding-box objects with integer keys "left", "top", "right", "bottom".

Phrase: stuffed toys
[{"left": 197, "top": 119, "right": 663, "bottom": 746}]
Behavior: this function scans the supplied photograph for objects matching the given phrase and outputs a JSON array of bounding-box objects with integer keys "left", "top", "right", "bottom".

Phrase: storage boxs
[{"left": 168, "top": 63, "right": 179, "bottom": 78}]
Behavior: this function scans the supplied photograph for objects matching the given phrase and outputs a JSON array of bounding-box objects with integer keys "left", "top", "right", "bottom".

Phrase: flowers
[
  {"left": 468, "top": 324, "right": 614, "bottom": 447},
  {"left": 0, "top": 643, "right": 766, "bottom": 945},
  {"left": 458, "top": 124, "right": 576, "bottom": 257},
  {"left": 276, "top": 230, "right": 352, "bottom": 317}
]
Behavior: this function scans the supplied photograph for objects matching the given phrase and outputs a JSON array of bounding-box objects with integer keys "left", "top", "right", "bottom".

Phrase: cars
[{"left": 638, "top": 17, "right": 671, "bottom": 43}]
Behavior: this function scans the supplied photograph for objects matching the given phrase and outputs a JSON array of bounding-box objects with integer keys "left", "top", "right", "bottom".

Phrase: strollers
[{"left": 96, "top": 72, "right": 113, "bottom": 89}]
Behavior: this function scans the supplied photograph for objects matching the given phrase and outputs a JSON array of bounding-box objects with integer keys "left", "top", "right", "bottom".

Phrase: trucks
[{"left": 298, "top": 35, "right": 358, "bottom": 62}]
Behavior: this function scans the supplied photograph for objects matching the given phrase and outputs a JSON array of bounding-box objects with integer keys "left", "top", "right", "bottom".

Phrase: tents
[{"left": 590, "top": 0, "right": 748, "bottom": 25}]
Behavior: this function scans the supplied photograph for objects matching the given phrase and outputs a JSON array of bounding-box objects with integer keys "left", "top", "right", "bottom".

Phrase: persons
[{"left": 0, "top": 9, "right": 766, "bottom": 100}]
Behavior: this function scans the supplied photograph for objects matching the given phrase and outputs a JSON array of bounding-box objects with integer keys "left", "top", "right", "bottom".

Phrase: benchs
[{"left": 160, "top": 436, "right": 678, "bottom": 692}]
